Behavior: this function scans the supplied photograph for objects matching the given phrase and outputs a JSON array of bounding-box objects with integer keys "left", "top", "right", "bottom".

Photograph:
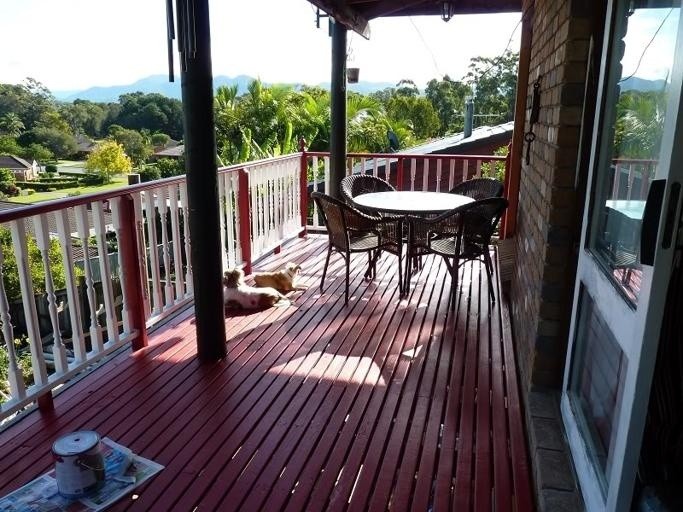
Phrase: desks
[{"left": 606, "top": 199, "right": 647, "bottom": 221}]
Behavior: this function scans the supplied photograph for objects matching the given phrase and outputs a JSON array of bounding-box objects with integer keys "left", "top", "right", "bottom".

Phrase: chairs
[
  {"left": 600, "top": 213, "right": 637, "bottom": 286},
  {"left": 311, "top": 175, "right": 508, "bottom": 313}
]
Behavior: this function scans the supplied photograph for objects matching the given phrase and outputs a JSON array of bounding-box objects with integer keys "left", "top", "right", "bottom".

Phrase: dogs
[
  {"left": 253, "top": 262, "right": 309, "bottom": 291},
  {"left": 223, "top": 267, "right": 299, "bottom": 311}
]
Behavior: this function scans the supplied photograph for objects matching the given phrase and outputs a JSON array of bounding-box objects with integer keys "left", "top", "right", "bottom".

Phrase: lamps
[{"left": 437, "top": 0, "right": 456, "bottom": 21}]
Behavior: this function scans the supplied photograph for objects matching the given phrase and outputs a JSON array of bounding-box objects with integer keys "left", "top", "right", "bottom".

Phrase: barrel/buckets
[{"left": 52, "top": 431, "right": 104, "bottom": 501}]
